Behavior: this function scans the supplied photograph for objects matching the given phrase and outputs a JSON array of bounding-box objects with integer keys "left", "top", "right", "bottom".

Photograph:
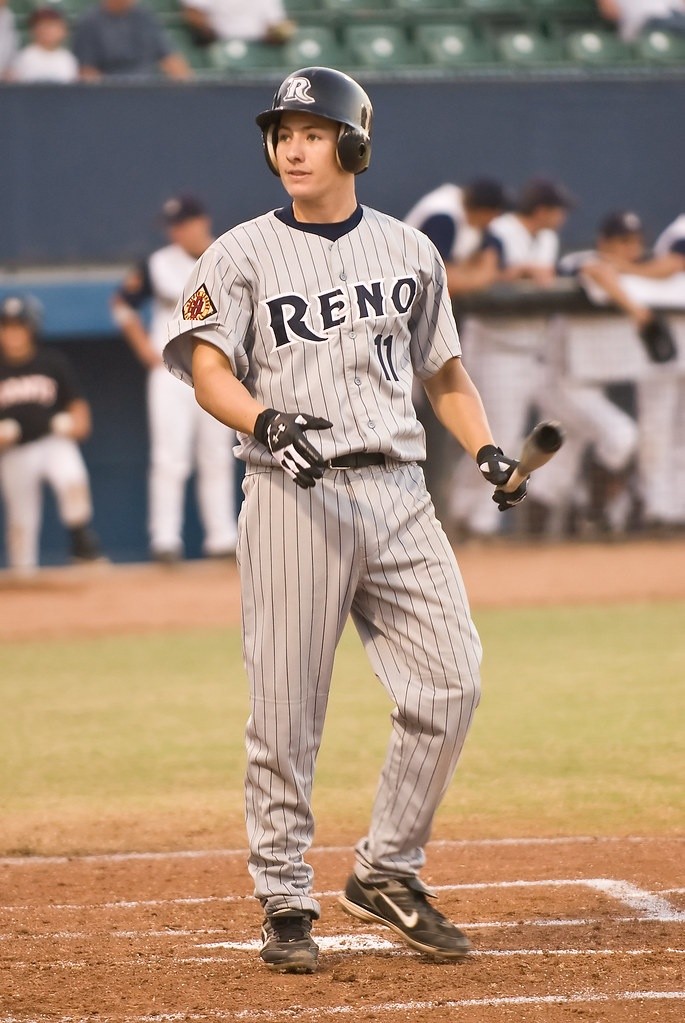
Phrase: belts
[{"left": 323, "top": 452, "right": 385, "bottom": 467}]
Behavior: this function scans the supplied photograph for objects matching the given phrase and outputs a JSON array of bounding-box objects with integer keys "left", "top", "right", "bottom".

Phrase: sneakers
[
  {"left": 260, "top": 907, "right": 319, "bottom": 973},
  {"left": 337, "top": 872, "right": 472, "bottom": 959}
]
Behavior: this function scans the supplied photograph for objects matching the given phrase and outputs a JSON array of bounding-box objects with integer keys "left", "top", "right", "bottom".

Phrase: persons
[
  {"left": 0, "top": 0, "right": 302, "bottom": 85},
  {"left": 0, "top": 294, "right": 104, "bottom": 575},
  {"left": 160, "top": 65, "right": 532, "bottom": 973},
  {"left": 108, "top": 175, "right": 685, "bottom": 569},
  {"left": 596, "top": 0, "right": 685, "bottom": 45}
]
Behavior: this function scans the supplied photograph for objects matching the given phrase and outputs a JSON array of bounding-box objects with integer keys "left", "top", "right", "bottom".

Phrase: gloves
[
  {"left": 254, "top": 408, "right": 333, "bottom": 489},
  {"left": 477, "top": 445, "right": 530, "bottom": 511}
]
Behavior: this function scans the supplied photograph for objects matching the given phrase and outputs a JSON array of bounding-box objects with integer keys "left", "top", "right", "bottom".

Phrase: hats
[
  {"left": 465, "top": 175, "right": 524, "bottom": 212},
  {"left": 604, "top": 211, "right": 642, "bottom": 237},
  {"left": 0, "top": 293, "right": 46, "bottom": 332},
  {"left": 524, "top": 174, "right": 577, "bottom": 211},
  {"left": 160, "top": 195, "right": 206, "bottom": 227}
]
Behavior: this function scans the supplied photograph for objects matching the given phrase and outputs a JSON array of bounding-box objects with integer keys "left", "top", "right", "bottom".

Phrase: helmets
[{"left": 255, "top": 66, "right": 374, "bottom": 177}]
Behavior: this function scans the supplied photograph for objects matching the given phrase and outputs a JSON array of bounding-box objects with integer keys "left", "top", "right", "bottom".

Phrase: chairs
[{"left": 0, "top": 0, "right": 685, "bottom": 77}]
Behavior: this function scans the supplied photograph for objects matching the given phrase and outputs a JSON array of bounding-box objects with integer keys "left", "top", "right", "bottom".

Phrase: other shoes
[{"left": 67, "top": 525, "right": 109, "bottom": 565}]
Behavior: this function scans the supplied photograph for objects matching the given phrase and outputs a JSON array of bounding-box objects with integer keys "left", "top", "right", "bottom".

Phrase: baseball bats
[{"left": 494, "top": 421, "right": 564, "bottom": 493}]
